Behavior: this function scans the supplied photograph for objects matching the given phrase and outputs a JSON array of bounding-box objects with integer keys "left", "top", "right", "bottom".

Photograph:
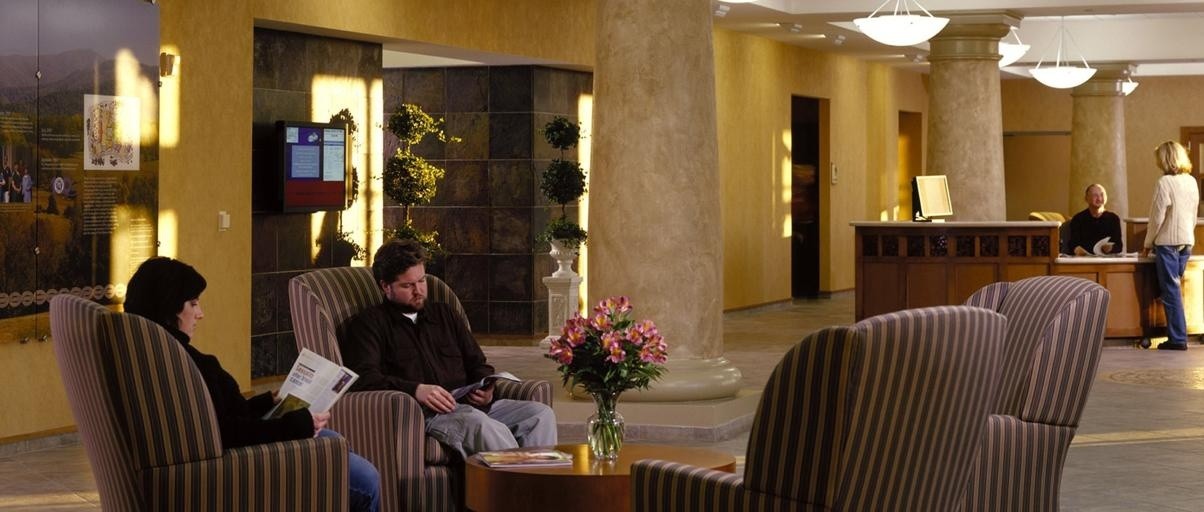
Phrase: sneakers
[{"left": 1157, "top": 341, "right": 1188, "bottom": 351}]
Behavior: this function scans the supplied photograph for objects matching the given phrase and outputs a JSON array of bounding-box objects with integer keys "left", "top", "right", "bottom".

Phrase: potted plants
[{"left": 537, "top": 112, "right": 589, "bottom": 279}]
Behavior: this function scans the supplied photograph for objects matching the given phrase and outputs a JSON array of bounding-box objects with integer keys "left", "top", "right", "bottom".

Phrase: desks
[
  {"left": 1124, "top": 217, "right": 1203, "bottom": 254},
  {"left": 849, "top": 218, "right": 1204, "bottom": 341}
]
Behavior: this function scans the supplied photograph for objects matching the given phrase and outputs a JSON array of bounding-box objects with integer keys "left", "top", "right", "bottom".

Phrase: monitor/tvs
[{"left": 911, "top": 174, "right": 953, "bottom": 222}]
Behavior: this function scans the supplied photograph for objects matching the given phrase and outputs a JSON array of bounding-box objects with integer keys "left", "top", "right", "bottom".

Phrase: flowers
[{"left": 548, "top": 294, "right": 668, "bottom": 458}]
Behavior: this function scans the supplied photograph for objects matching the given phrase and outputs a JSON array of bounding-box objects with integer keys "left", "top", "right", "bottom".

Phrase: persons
[
  {"left": 122, "top": 256, "right": 383, "bottom": 511},
  {"left": 1066, "top": 183, "right": 1122, "bottom": 253},
  {"left": 1, "top": 160, "right": 32, "bottom": 202},
  {"left": 337, "top": 237, "right": 558, "bottom": 462},
  {"left": 1140, "top": 140, "right": 1199, "bottom": 350}
]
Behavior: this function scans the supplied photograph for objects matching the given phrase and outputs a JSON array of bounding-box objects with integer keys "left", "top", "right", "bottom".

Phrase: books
[
  {"left": 261, "top": 346, "right": 359, "bottom": 442},
  {"left": 451, "top": 371, "right": 522, "bottom": 398},
  {"left": 476, "top": 448, "right": 573, "bottom": 468},
  {"left": 1080, "top": 237, "right": 1111, "bottom": 256}
]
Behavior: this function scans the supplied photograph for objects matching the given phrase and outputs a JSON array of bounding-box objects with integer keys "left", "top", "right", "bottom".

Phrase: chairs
[
  {"left": 289, "top": 266, "right": 556, "bottom": 512},
  {"left": 963, "top": 276, "right": 1110, "bottom": 511},
  {"left": 1030, "top": 209, "right": 1072, "bottom": 226},
  {"left": 631, "top": 306, "right": 1015, "bottom": 510},
  {"left": 51, "top": 296, "right": 349, "bottom": 511}
]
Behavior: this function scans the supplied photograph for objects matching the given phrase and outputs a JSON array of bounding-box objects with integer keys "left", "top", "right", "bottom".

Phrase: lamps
[
  {"left": 852, "top": 0, "right": 950, "bottom": 48},
  {"left": 1029, "top": 15, "right": 1098, "bottom": 89},
  {"left": 999, "top": 27, "right": 1031, "bottom": 68},
  {"left": 1115, "top": 77, "right": 1140, "bottom": 96}
]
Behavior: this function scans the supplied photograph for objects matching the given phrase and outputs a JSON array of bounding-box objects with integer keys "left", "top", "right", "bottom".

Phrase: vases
[{"left": 586, "top": 389, "right": 626, "bottom": 461}]
